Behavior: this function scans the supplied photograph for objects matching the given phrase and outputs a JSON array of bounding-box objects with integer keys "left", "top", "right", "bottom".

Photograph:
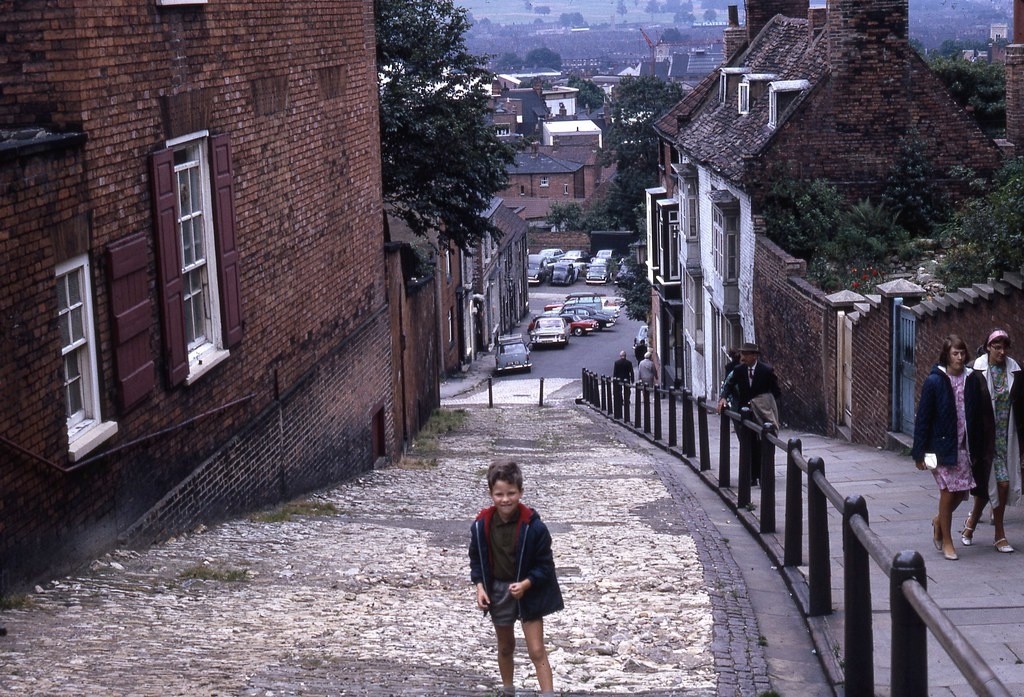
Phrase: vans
[{"left": 528, "top": 254, "right": 547, "bottom": 287}]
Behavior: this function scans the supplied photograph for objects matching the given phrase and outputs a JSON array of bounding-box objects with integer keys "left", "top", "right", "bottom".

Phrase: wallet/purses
[{"left": 924, "top": 453, "right": 938, "bottom": 469}]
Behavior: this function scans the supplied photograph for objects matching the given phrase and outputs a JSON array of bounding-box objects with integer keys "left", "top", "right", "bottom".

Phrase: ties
[{"left": 748, "top": 368, "right": 754, "bottom": 380}]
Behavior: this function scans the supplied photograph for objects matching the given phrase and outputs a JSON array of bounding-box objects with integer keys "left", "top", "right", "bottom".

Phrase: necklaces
[{"left": 991, "top": 374, "right": 1004, "bottom": 392}]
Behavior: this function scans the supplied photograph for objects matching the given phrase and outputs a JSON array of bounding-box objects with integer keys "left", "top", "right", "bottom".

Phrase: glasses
[{"left": 989, "top": 343, "right": 1011, "bottom": 351}]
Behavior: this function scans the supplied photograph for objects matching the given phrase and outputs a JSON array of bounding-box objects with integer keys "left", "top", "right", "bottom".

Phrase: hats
[{"left": 738, "top": 343, "right": 761, "bottom": 352}]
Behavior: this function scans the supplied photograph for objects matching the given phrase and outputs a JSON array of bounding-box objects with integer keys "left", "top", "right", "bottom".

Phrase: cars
[
  {"left": 527, "top": 292, "right": 624, "bottom": 337},
  {"left": 495, "top": 334, "right": 533, "bottom": 373},
  {"left": 634, "top": 326, "right": 648, "bottom": 350},
  {"left": 538, "top": 249, "right": 638, "bottom": 286},
  {"left": 529, "top": 317, "right": 572, "bottom": 350}
]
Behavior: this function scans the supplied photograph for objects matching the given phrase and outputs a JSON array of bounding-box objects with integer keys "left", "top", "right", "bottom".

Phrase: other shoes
[
  {"left": 750, "top": 478, "right": 760, "bottom": 485},
  {"left": 994, "top": 539, "right": 1015, "bottom": 552},
  {"left": 495, "top": 688, "right": 515, "bottom": 697},
  {"left": 960, "top": 517, "right": 975, "bottom": 545},
  {"left": 990, "top": 512, "right": 994, "bottom": 524}
]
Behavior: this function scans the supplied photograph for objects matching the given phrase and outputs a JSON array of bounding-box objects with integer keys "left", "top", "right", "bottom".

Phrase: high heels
[
  {"left": 941, "top": 543, "right": 959, "bottom": 560},
  {"left": 932, "top": 516, "right": 943, "bottom": 550}
]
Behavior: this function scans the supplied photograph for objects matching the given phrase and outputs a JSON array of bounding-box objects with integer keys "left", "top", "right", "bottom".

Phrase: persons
[
  {"left": 963, "top": 328, "right": 1024, "bottom": 553},
  {"left": 718, "top": 343, "right": 782, "bottom": 486},
  {"left": 912, "top": 334, "right": 980, "bottom": 559},
  {"left": 638, "top": 352, "right": 657, "bottom": 404},
  {"left": 613, "top": 351, "right": 634, "bottom": 405},
  {"left": 469, "top": 460, "right": 564, "bottom": 697},
  {"left": 635, "top": 340, "right": 647, "bottom": 366}
]
